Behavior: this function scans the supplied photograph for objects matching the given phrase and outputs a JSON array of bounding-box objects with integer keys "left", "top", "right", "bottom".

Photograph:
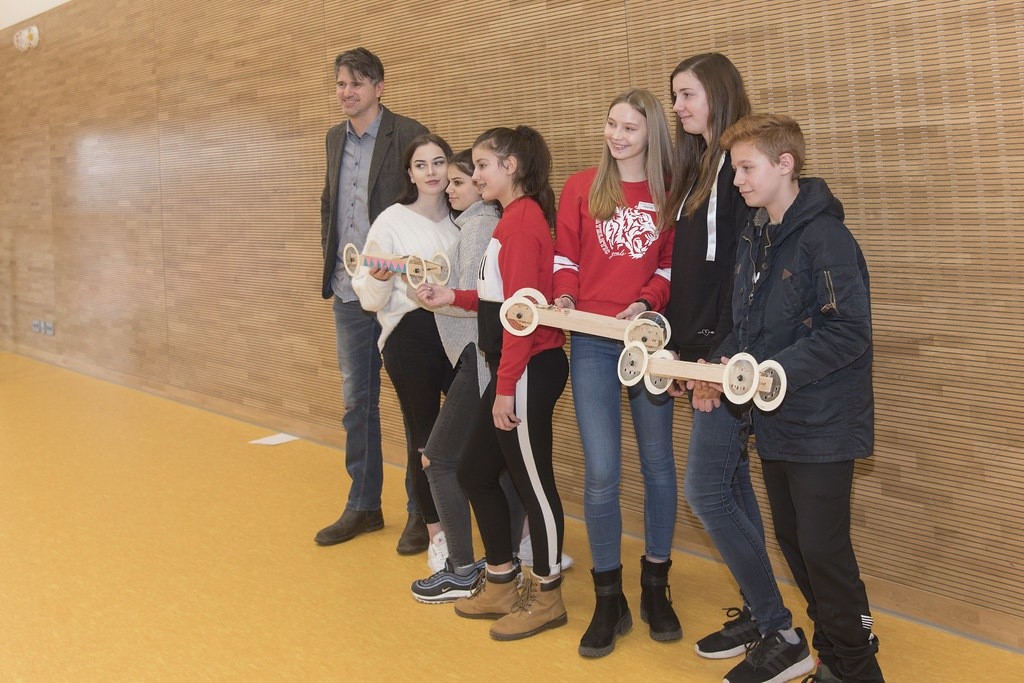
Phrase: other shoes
[{"left": 800, "top": 652, "right": 885, "bottom": 683}]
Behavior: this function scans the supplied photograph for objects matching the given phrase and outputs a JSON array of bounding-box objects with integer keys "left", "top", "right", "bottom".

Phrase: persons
[
  {"left": 665, "top": 52, "right": 814, "bottom": 683},
  {"left": 351, "top": 135, "right": 573, "bottom": 577},
  {"left": 686, "top": 114, "right": 885, "bottom": 683},
  {"left": 553, "top": 87, "right": 683, "bottom": 660},
  {"left": 454, "top": 127, "right": 570, "bottom": 643},
  {"left": 410, "top": 148, "right": 499, "bottom": 604},
  {"left": 314, "top": 47, "right": 431, "bottom": 556}
]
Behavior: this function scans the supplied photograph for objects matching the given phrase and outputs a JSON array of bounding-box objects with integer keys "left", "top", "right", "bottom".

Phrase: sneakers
[
  {"left": 426, "top": 530, "right": 450, "bottom": 573},
  {"left": 723, "top": 626, "right": 816, "bottom": 683},
  {"left": 518, "top": 535, "right": 575, "bottom": 570},
  {"left": 694, "top": 605, "right": 792, "bottom": 658},
  {"left": 472, "top": 555, "right": 523, "bottom": 581},
  {"left": 410, "top": 558, "right": 482, "bottom": 605}
]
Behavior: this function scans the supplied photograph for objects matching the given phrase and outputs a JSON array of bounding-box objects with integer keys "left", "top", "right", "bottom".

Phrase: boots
[
  {"left": 453, "top": 564, "right": 522, "bottom": 619},
  {"left": 488, "top": 569, "right": 568, "bottom": 641},
  {"left": 640, "top": 554, "right": 682, "bottom": 641},
  {"left": 577, "top": 564, "right": 633, "bottom": 656}
]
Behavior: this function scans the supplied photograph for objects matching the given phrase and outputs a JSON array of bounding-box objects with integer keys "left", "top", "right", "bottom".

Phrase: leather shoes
[
  {"left": 314, "top": 509, "right": 385, "bottom": 545},
  {"left": 395, "top": 514, "right": 431, "bottom": 552}
]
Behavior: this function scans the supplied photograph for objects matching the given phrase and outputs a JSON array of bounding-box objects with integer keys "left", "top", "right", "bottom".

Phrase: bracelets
[
  {"left": 561, "top": 296, "right": 576, "bottom": 307},
  {"left": 635, "top": 297, "right": 651, "bottom": 312}
]
